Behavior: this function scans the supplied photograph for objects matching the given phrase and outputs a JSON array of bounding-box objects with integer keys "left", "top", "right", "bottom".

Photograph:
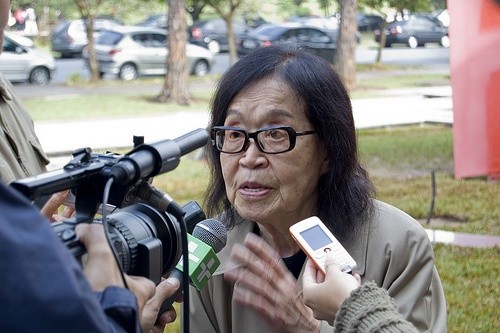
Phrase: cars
[
  {"left": 186, "top": 15, "right": 268, "bottom": 52},
  {"left": 80, "top": 27, "right": 216, "bottom": 83},
  {"left": 236, "top": 23, "right": 339, "bottom": 65},
  {"left": 285, "top": 16, "right": 362, "bottom": 48},
  {"left": 375, "top": 14, "right": 451, "bottom": 49},
  {"left": 0, "top": 28, "right": 56, "bottom": 87},
  {"left": 136, "top": 13, "right": 186, "bottom": 35},
  {"left": 328, "top": 14, "right": 396, "bottom": 48},
  {"left": 52, "top": 19, "right": 127, "bottom": 57}
]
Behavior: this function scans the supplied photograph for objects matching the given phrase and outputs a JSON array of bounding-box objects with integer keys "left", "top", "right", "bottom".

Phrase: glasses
[{"left": 209, "top": 126, "right": 318, "bottom": 154}]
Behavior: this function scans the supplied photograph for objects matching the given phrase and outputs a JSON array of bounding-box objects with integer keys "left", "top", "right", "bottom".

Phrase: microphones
[{"left": 154, "top": 220, "right": 228, "bottom": 325}]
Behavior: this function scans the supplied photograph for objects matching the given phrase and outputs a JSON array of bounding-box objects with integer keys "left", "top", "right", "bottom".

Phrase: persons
[
  {"left": 302, "top": 256, "right": 419, "bottom": 333},
  {"left": 175, "top": 44, "right": 448, "bottom": 333},
  {"left": 0, "top": 0, "right": 184, "bottom": 333}
]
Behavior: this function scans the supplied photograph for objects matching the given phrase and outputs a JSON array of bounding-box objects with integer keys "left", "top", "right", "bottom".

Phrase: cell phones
[{"left": 289, "top": 215, "right": 357, "bottom": 279}]
